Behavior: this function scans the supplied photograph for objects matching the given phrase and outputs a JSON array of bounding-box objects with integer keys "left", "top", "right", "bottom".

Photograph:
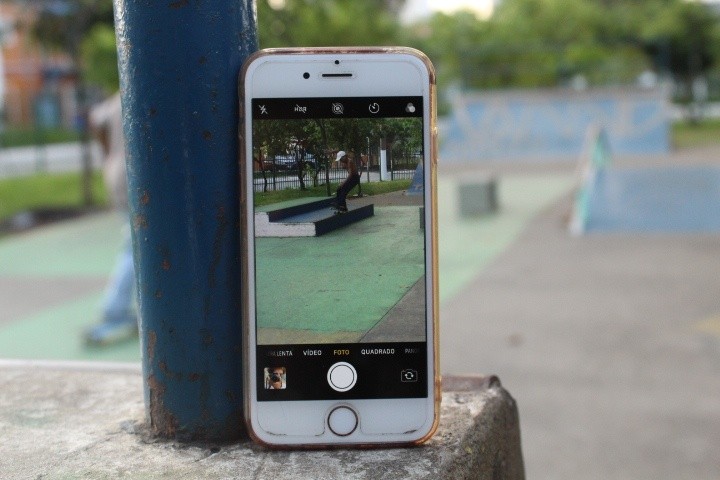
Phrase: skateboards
[{"left": 333, "top": 204, "right": 348, "bottom": 214}]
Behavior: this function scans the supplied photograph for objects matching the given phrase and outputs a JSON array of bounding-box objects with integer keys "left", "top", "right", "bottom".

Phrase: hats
[{"left": 335, "top": 151, "right": 346, "bottom": 161}]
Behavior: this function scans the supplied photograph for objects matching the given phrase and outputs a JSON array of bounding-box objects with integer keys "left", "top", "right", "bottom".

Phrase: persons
[
  {"left": 332, "top": 149, "right": 360, "bottom": 210},
  {"left": 81, "top": 83, "right": 139, "bottom": 344}
]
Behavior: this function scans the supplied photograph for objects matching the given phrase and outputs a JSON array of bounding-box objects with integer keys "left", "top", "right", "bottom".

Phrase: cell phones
[{"left": 241, "top": 42, "right": 444, "bottom": 448}]
